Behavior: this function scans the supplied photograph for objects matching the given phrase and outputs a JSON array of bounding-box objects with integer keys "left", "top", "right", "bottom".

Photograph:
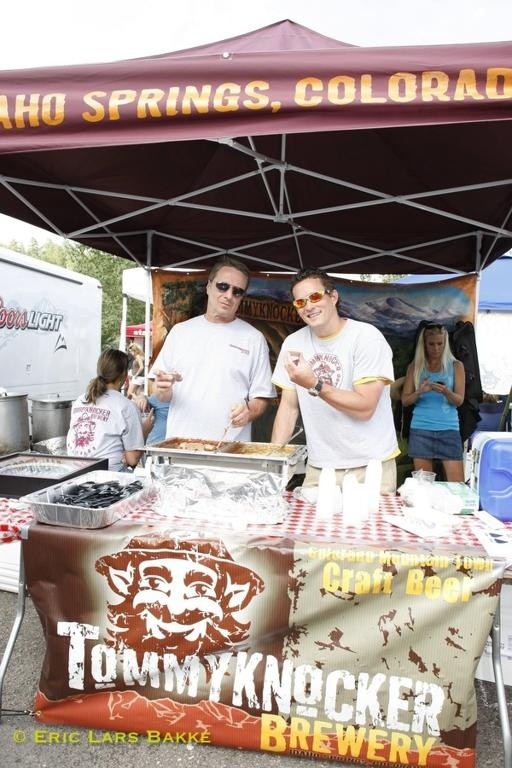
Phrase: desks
[{"left": 0, "top": 454, "right": 511, "bottom": 768}]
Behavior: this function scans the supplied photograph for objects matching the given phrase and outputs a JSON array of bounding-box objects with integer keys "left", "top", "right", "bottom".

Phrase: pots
[
  {"left": 28, "top": 391, "right": 78, "bottom": 441},
  {"left": 0, "top": 391, "right": 28, "bottom": 456}
]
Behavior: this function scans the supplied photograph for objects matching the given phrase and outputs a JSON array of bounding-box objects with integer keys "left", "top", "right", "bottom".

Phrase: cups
[
  {"left": 413, "top": 469, "right": 436, "bottom": 485},
  {"left": 140, "top": 413, "right": 155, "bottom": 424}
]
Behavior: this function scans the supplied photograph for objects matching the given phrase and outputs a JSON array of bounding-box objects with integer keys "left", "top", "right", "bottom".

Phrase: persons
[
  {"left": 146, "top": 258, "right": 278, "bottom": 442},
  {"left": 146, "top": 394, "right": 170, "bottom": 445},
  {"left": 401, "top": 323, "right": 466, "bottom": 482},
  {"left": 127, "top": 343, "right": 145, "bottom": 400},
  {"left": 270, "top": 266, "right": 401, "bottom": 496},
  {"left": 66, "top": 347, "right": 155, "bottom": 472}
]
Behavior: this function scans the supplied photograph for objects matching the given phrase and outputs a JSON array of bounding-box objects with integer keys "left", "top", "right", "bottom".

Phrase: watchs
[{"left": 307, "top": 378, "right": 323, "bottom": 397}]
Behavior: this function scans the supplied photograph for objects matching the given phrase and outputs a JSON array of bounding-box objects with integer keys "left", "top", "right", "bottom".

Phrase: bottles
[{"left": 317, "top": 456, "right": 384, "bottom": 522}]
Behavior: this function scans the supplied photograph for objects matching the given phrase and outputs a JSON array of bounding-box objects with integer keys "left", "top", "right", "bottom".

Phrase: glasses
[
  {"left": 293, "top": 290, "right": 325, "bottom": 308},
  {"left": 213, "top": 278, "right": 245, "bottom": 298},
  {"left": 424, "top": 324, "right": 444, "bottom": 331}
]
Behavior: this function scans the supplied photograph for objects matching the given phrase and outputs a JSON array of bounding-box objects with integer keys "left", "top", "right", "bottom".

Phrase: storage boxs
[{"left": 468, "top": 429, "right": 511, "bottom": 523}]
